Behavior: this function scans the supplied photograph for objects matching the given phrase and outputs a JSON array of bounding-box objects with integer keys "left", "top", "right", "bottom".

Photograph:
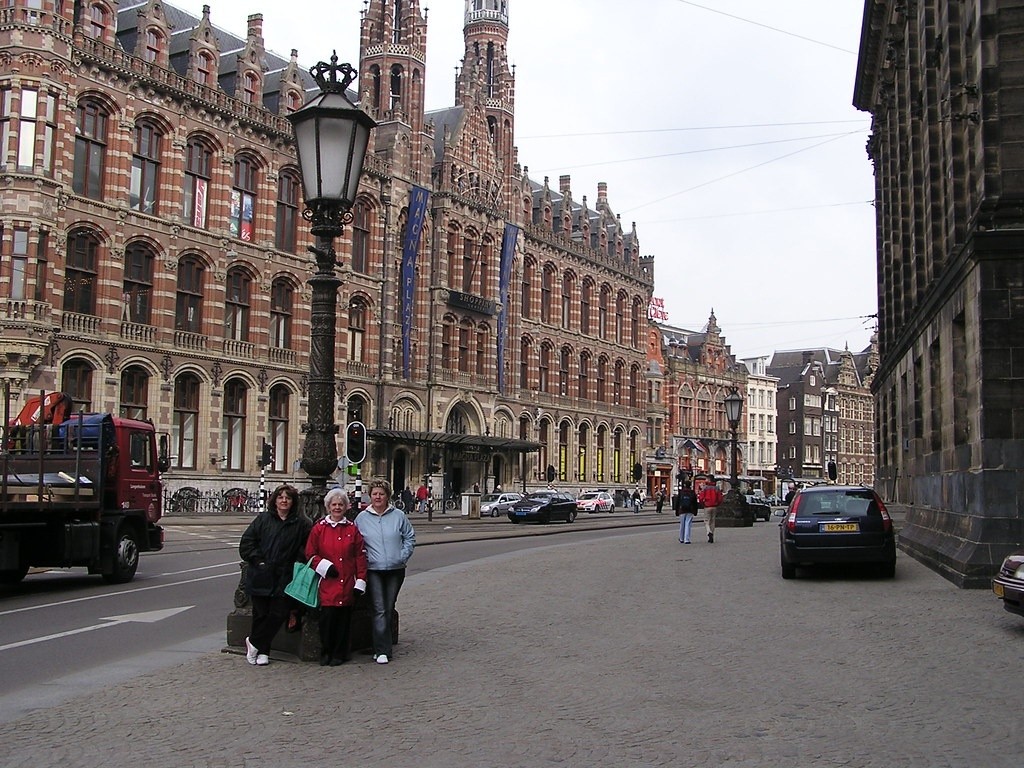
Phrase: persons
[
  {"left": 492, "top": 484, "right": 502, "bottom": 493},
  {"left": 283, "top": 487, "right": 367, "bottom": 666},
  {"left": 785, "top": 491, "right": 801, "bottom": 506},
  {"left": 238, "top": 485, "right": 312, "bottom": 664},
  {"left": 746, "top": 487, "right": 754, "bottom": 495},
  {"left": 402, "top": 484, "right": 428, "bottom": 514},
  {"left": 473, "top": 482, "right": 480, "bottom": 493},
  {"left": 352, "top": 479, "right": 416, "bottom": 664},
  {"left": 697, "top": 475, "right": 723, "bottom": 543},
  {"left": 675, "top": 481, "right": 697, "bottom": 544}
]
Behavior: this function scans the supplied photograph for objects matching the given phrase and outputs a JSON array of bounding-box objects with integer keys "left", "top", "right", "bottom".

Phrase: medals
[{"left": 621, "top": 488, "right": 666, "bottom": 513}]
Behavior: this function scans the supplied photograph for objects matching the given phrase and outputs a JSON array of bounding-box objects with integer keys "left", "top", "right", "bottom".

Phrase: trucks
[{"left": 0, "top": 381, "right": 171, "bottom": 592}]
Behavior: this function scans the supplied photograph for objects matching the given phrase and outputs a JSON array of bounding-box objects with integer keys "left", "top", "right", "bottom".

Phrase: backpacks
[{"left": 680, "top": 490, "right": 693, "bottom": 510}]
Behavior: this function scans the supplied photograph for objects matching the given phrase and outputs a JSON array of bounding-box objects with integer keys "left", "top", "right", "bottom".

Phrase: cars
[
  {"left": 754, "top": 489, "right": 782, "bottom": 506},
  {"left": 575, "top": 492, "right": 615, "bottom": 513},
  {"left": 480, "top": 493, "right": 522, "bottom": 517},
  {"left": 774, "top": 484, "right": 897, "bottom": 579},
  {"left": 990, "top": 547, "right": 1024, "bottom": 616},
  {"left": 507, "top": 491, "right": 577, "bottom": 523},
  {"left": 745, "top": 495, "right": 771, "bottom": 521},
  {"left": 347, "top": 492, "right": 368, "bottom": 512}
]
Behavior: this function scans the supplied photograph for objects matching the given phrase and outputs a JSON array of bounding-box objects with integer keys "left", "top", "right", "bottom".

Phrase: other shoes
[
  {"left": 373, "top": 653, "right": 389, "bottom": 663},
  {"left": 708, "top": 532, "right": 713, "bottom": 543},
  {"left": 679, "top": 539, "right": 691, "bottom": 544},
  {"left": 327, "top": 657, "right": 343, "bottom": 666}
]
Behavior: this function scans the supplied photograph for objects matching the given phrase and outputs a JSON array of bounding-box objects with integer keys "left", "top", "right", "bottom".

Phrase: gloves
[
  {"left": 327, "top": 565, "right": 338, "bottom": 577},
  {"left": 354, "top": 588, "right": 364, "bottom": 594}
]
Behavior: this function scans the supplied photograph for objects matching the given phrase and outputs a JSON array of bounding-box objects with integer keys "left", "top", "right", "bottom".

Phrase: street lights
[
  {"left": 223, "top": 53, "right": 401, "bottom": 652},
  {"left": 715, "top": 384, "right": 754, "bottom": 527},
  {"left": 821, "top": 388, "right": 839, "bottom": 478}
]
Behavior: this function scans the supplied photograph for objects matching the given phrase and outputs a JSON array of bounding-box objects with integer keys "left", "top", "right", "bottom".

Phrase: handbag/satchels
[{"left": 283, "top": 555, "right": 322, "bottom": 608}]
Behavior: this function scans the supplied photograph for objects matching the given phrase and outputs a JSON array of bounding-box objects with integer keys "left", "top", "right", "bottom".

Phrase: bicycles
[
  {"left": 166, "top": 490, "right": 259, "bottom": 512},
  {"left": 446, "top": 493, "right": 462, "bottom": 510}
]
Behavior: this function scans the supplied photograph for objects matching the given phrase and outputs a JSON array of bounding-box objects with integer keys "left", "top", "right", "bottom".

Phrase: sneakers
[{"left": 246, "top": 636, "right": 269, "bottom": 665}]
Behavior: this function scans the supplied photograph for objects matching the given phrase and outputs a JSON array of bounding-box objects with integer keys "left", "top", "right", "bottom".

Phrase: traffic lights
[
  {"left": 265, "top": 443, "right": 275, "bottom": 465},
  {"left": 828, "top": 463, "right": 837, "bottom": 480},
  {"left": 346, "top": 421, "right": 367, "bottom": 464},
  {"left": 433, "top": 453, "right": 442, "bottom": 473}
]
